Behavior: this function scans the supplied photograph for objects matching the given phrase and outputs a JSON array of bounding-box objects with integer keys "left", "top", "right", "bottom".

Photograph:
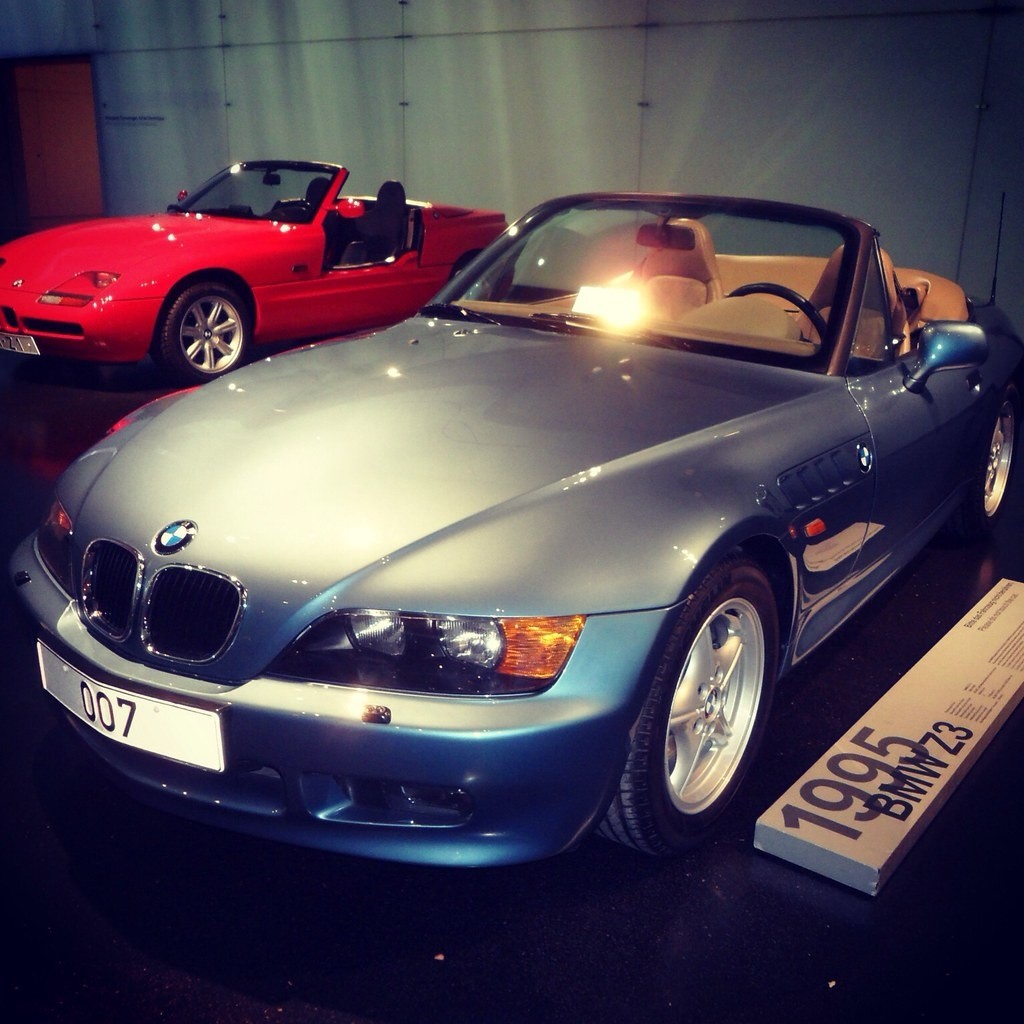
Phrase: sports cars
[
  {"left": 0, "top": 158, "right": 517, "bottom": 390},
  {"left": 11, "top": 188, "right": 1024, "bottom": 876}
]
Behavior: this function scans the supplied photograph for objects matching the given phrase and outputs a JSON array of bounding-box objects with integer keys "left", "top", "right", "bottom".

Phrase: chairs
[
  {"left": 615, "top": 216, "right": 724, "bottom": 327},
  {"left": 361, "top": 180, "right": 407, "bottom": 261},
  {"left": 796, "top": 239, "right": 909, "bottom": 362},
  {"left": 304, "top": 174, "right": 337, "bottom": 238}
]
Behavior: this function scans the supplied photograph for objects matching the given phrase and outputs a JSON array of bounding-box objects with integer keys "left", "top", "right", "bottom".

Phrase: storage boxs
[{"left": 752, "top": 573, "right": 1024, "bottom": 900}]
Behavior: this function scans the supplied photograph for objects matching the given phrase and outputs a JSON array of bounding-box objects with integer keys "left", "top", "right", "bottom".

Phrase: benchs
[{"left": 710, "top": 251, "right": 970, "bottom": 346}]
